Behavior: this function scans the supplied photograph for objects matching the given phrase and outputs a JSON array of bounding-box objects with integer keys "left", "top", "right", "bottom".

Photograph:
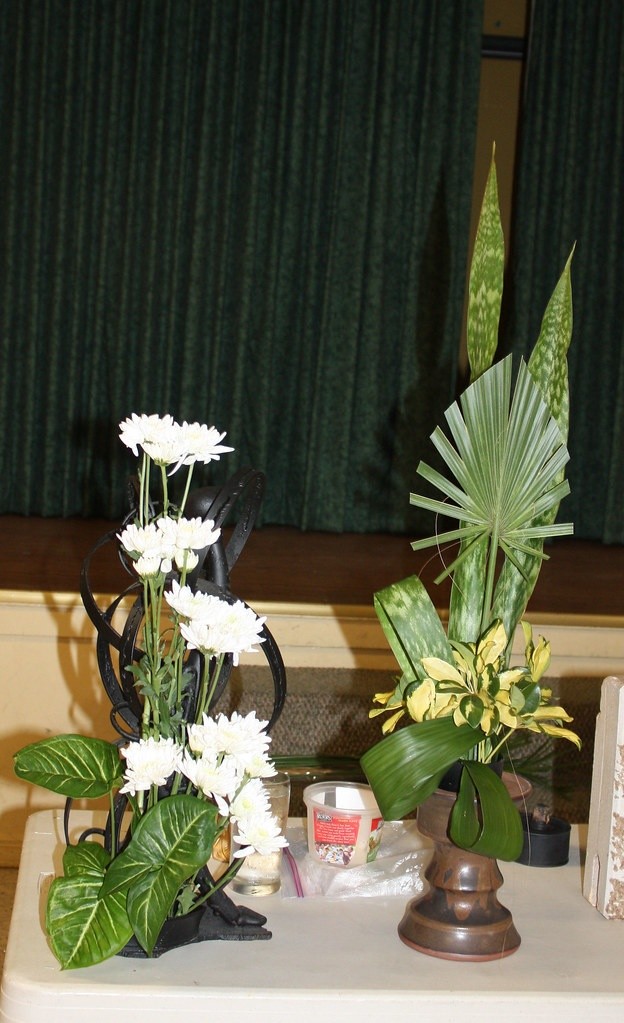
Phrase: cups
[
  {"left": 230, "top": 769, "right": 291, "bottom": 897},
  {"left": 303, "top": 781, "right": 384, "bottom": 869}
]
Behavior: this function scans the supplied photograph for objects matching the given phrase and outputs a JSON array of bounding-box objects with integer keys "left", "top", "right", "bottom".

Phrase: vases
[
  {"left": 399, "top": 771, "right": 531, "bottom": 960},
  {"left": 120, "top": 772, "right": 273, "bottom": 959}
]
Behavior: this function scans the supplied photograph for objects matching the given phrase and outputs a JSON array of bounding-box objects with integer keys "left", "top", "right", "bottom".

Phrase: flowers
[
  {"left": 11, "top": 416, "right": 291, "bottom": 970},
  {"left": 361, "top": 353, "right": 582, "bottom": 863}
]
